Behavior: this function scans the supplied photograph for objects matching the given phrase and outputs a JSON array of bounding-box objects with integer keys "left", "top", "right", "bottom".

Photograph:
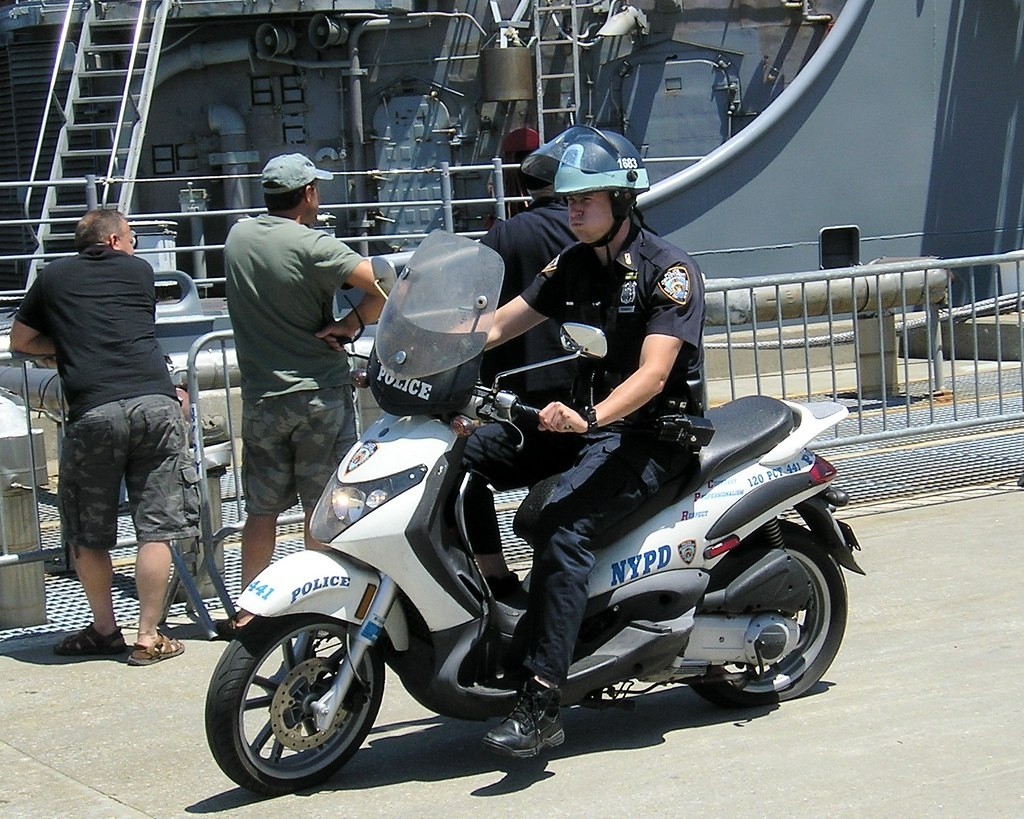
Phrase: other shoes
[{"left": 215, "top": 611, "right": 247, "bottom": 642}]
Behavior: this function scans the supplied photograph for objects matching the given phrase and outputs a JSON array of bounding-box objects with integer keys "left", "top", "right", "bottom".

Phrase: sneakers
[{"left": 481, "top": 679, "right": 565, "bottom": 759}]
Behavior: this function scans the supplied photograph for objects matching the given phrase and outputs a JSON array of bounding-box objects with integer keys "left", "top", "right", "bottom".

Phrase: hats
[
  {"left": 517, "top": 154, "right": 554, "bottom": 190},
  {"left": 260, "top": 152, "right": 335, "bottom": 196}
]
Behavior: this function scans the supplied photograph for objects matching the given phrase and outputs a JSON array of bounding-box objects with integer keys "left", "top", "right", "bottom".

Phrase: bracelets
[{"left": 579, "top": 405, "right": 598, "bottom": 431}]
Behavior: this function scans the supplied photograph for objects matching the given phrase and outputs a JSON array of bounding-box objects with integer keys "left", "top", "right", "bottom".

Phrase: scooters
[{"left": 204, "top": 227, "right": 866, "bottom": 797}]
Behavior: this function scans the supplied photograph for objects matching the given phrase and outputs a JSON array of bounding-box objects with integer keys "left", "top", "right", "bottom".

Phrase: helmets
[{"left": 555, "top": 130, "right": 650, "bottom": 197}]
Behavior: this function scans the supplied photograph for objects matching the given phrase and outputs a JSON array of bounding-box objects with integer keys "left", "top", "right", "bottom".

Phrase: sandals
[
  {"left": 128, "top": 626, "right": 185, "bottom": 664},
  {"left": 54, "top": 622, "right": 129, "bottom": 657}
]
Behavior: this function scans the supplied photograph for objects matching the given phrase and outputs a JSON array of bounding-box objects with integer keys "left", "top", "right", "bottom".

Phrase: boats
[{"left": 0, "top": 1, "right": 1024, "bottom": 358}]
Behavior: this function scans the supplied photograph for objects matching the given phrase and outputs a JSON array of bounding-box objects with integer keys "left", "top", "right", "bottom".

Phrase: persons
[
  {"left": 11, "top": 208, "right": 202, "bottom": 665},
  {"left": 473, "top": 165, "right": 580, "bottom": 409},
  {"left": 222, "top": 152, "right": 386, "bottom": 627},
  {"left": 449, "top": 125, "right": 705, "bottom": 758}
]
